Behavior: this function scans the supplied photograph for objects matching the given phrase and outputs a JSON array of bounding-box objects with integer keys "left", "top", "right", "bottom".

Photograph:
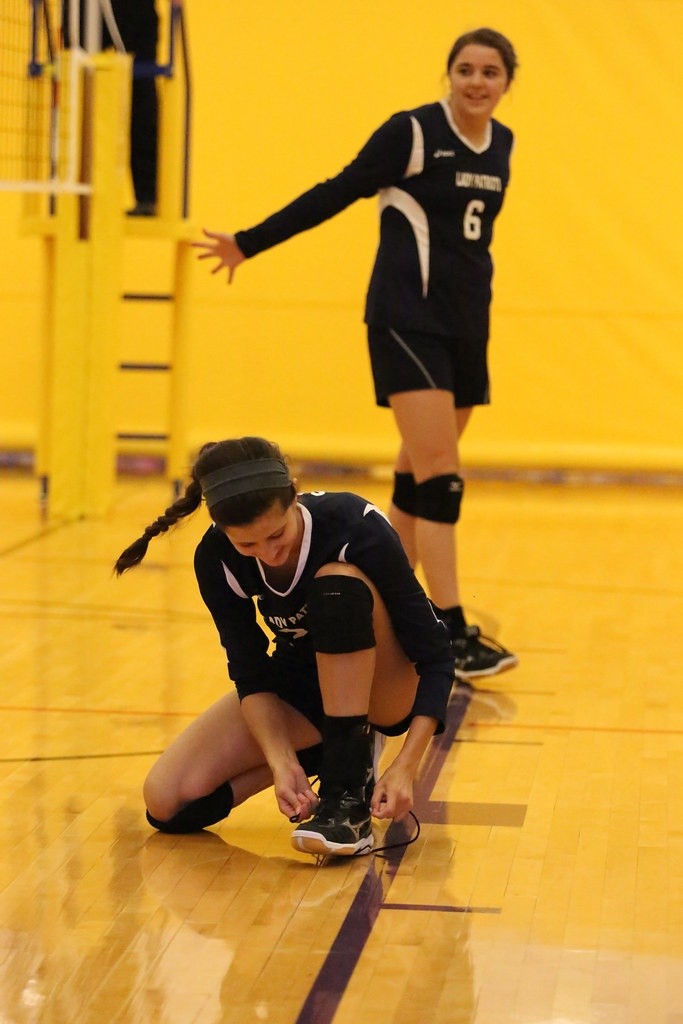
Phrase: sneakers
[
  {"left": 359, "top": 726, "right": 388, "bottom": 813},
  {"left": 291, "top": 784, "right": 375, "bottom": 857},
  {"left": 450, "top": 624, "right": 518, "bottom": 679}
]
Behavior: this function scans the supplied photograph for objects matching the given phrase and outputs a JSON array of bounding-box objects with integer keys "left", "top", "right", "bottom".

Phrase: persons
[
  {"left": 58, "top": 0, "right": 162, "bottom": 217},
  {"left": 185, "top": 21, "right": 519, "bottom": 682},
  {"left": 113, "top": 435, "right": 461, "bottom": 862}
]
